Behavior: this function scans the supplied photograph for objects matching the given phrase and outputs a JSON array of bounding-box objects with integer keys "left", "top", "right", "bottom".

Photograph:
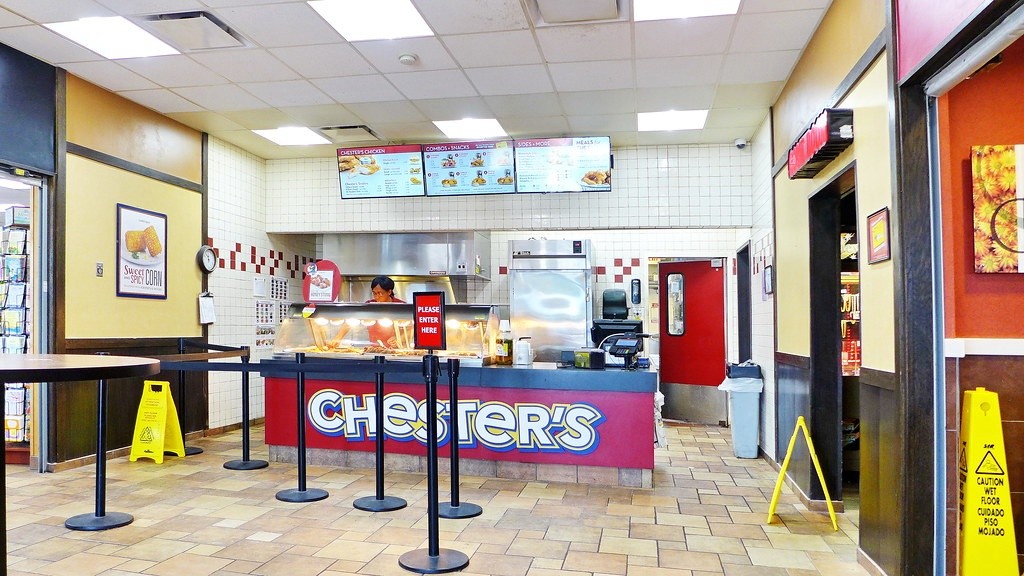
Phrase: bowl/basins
[{"left": 577, "top": 179, "right": 610, "bottom": 191}]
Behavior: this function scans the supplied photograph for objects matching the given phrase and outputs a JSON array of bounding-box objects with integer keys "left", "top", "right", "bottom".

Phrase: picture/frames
[
  {"left": 116, "top": 203, "right": 168, "bottom": 300},
  {"left": 867, "top": 206, "right": 891, "bottom": 266},
  {"left": 764, "top": 265, "right": 773, "bottom": 294}
]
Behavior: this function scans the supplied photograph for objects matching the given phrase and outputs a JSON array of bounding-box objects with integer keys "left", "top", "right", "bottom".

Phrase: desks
[{"left": 0, "top": 354, "right": 160, "bottom": 576}]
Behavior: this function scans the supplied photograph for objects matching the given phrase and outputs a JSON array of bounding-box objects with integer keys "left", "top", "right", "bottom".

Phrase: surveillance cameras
[{"left": 735, "top": 139, "right": 746, "bottom": 149}]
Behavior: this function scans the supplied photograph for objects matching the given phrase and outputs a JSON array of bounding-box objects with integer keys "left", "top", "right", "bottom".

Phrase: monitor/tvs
[{"left": 590, "top": 319, "right": 644, "bottom": 352}]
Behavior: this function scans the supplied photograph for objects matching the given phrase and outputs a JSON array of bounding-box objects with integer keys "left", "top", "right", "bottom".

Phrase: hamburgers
[{"left": 409, "top": 156, "right": 419, "bottom": 164}]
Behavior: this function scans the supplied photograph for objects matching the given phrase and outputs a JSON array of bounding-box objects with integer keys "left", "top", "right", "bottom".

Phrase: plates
[{"left": 122, "top": 238, "right": 162, "bottom": 266}]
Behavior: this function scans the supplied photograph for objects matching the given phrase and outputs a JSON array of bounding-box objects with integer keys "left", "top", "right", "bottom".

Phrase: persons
[{"left": 365, "top": 276, "right": 406, "bottom": 303}]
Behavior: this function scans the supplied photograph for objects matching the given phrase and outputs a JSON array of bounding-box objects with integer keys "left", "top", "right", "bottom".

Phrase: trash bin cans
[{"left": 725, "top": 361, "right": 763, "bottom": 459}]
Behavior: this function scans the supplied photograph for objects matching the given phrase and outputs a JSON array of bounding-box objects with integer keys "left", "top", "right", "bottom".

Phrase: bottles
[{"left": 495, "top": 319, "right": 513, "bottom": 367}]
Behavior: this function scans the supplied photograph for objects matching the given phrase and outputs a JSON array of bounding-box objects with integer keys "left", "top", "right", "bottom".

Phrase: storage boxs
[{"left": 5, "top": 206, "right": 30, "bottom": 228}]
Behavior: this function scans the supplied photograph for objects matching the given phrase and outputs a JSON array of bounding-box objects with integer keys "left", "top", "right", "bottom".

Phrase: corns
[
  {"left": 126, "top": 231, "right": 146, "bottom": 252},
  {"left": 144, "top": 226, "right": 162, "bottom": 257}
]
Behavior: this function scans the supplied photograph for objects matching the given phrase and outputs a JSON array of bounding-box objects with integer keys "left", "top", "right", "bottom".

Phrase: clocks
[{"left": 198, "top": 245, "right": 217, "bottom": 274}]
